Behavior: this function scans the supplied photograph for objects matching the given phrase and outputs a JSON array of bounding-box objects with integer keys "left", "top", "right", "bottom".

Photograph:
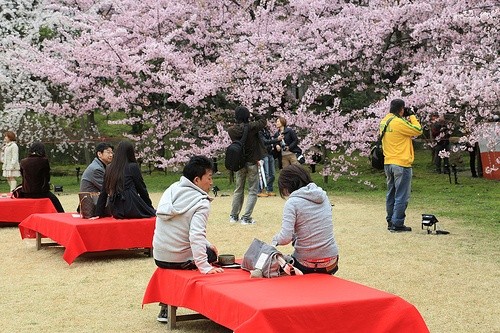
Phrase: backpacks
[{"left": 225, "top": 127, "right": 245, "bottom": 172}]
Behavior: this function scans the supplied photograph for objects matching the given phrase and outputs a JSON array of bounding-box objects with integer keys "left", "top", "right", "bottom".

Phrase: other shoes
[
  {"left": 230, "top": 215, "right": 238, "bottom": 222},
  {"left": 241, "top": 218, "right": 255, "bottom": 225},
  {"left": 257, "top": 193, "right": 268, "bottom": 197},
  {"left": 265, "top": 191, "right": 276, "bottom": 196},
  {"left": 157, "top": 310, "right": 168, "bottom": 322},
  {"left": 388, "top": 221, "right": 411, "bottom": 232}
]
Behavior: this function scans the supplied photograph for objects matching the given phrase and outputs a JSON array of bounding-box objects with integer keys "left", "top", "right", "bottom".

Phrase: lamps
[
  {"left": 421, "top": 212, "right": 439, "bottom": 230},
  {"left": 212, "top": 186, "right": 220, "bottom": 198},
  {"left": 55, "top": 185, "right": 64, "bottom": 196}
]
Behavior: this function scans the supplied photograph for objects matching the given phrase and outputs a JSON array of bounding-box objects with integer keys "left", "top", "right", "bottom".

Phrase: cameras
[{"left": 403, "top": 106, "right": 417, "bottom": 117}]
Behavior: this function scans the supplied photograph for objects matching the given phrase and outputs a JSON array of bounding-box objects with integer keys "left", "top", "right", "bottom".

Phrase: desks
[
  {"left": 142, "top": 258, "right": 429, "bottom": 333},
  {"left": 19, "top": 212, "right": 157, "bottom": 266},
  {"left": 0, "top": 194, "right": 58, "bottom": 228}
]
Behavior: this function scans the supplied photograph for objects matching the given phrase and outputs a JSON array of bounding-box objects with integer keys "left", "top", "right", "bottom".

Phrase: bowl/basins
[{"left": 218, "top": 254, "right": 235, "bottom": 265}]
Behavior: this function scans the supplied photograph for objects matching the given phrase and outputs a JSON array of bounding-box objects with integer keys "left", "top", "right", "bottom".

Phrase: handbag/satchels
[
  {"left": 10, "top": 184, "right": 22, "bottom": 199},
  {"left": 241, "top": 238, "right": 304, "bottom": 278},
  {"left": 78, "top": 191, "right": 112, "bottom": 219},
  {"left": 370, "top": 148, "right": 384, "bottom": 171}
]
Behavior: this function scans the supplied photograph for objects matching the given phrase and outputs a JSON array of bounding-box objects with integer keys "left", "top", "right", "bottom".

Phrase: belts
[{"left": 294, "top": 256, "right": 339, "bottom": 269}]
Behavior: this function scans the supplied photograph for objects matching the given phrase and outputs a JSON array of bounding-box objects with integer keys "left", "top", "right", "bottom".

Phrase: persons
[
  {"left": 273, "top": 118, "right": 302, "bottom": 169},
  {"left": 257, "top": 126, "right": 284, "bottom": 197},
  {"left": 20, "top": 142, "right": 65, "bottom": 213},
  {"left": 90, "top": 141, "right": 156, "bottom": 256},
  {"left": 271, "top": 164, "right": 339, "bottom": 275},
  {"left": 2, "top": 131, "right": 21, "bottom": 192},
  {"left": 467, "top": 141, "right": 483, "bottom": 177},
  {"left": 432, "top": 124, "right": 450, "bottom": 174},
  {"left": 380, "top": 99, "right": 423, "bottom": 233},
  {"left": 152, "top": 156, "right": 224, "bottom": 321},
  {"left": 228, "top": 106, "right": 267, "bottom": 225},
  {"left": 80, "top": 143, "right": 114, "bottom": 192}
]
